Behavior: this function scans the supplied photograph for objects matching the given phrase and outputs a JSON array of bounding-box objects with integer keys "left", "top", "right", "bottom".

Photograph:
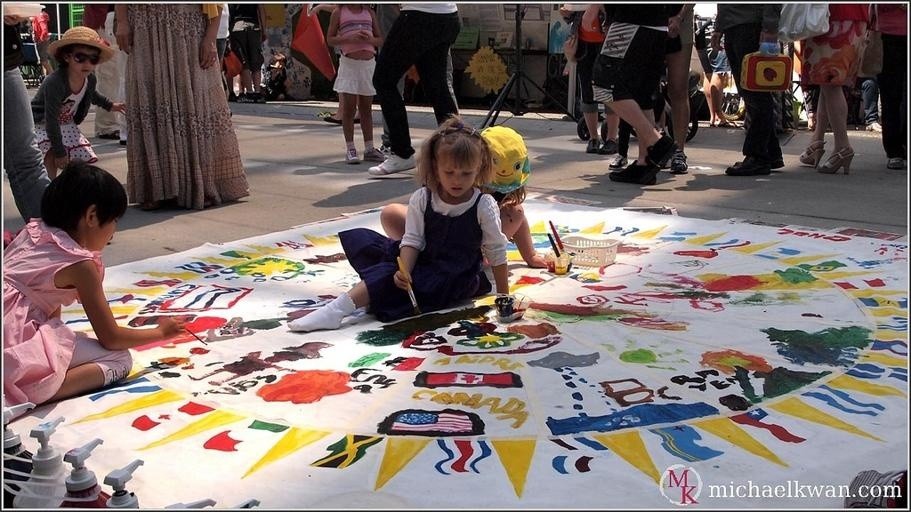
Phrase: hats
[
  {"left": 47, "top": 26, "right": 115, "bottom": 65},
  {"left": 476, "top": 126, "right": 531, "bottom": 194}
]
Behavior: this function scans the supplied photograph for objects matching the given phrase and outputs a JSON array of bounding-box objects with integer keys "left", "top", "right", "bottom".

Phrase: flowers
[{"left": 464, "top": 46, "right": 510, "bottom": 95}]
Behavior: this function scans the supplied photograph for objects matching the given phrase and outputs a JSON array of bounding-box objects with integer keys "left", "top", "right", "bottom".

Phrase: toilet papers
[{"left": 3, "top": 403, "right": 261, "bottom": 509}]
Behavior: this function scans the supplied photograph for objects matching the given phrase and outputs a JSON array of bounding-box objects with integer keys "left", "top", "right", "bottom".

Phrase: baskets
[{"left": 554, "top": 235, "right": 623, "bottom": 268}]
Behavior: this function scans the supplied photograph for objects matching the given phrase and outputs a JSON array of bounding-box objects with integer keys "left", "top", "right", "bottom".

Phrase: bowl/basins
[
  {"left": 495, "top": 293, "right": 532, "bottom": 322},
  {"left": 5, "top": 4, "right": 46, "bottom": 17}
]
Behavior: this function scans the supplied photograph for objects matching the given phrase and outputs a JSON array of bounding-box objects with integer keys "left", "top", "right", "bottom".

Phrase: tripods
[{"left": 481, "top": 4, "right": 578, "bottom": 129}]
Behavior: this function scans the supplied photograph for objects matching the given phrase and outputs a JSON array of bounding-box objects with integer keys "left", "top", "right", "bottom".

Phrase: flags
[{"left": 392, "top": 409, "right": 473, "bottom": 434}]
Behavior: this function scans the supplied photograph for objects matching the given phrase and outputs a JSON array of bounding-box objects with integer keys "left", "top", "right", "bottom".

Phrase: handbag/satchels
[
  {"left": 740, "top": 41, "right": 793, "bottom": 92},
  {"left": 856, "top": 29, "right": 884, "bottom": 78},
  {"left": 222, "top": 51, "right": 244, "bottom": 79},
  {"left": 777, "top": 3, "right": 831, "bottom": 42}
]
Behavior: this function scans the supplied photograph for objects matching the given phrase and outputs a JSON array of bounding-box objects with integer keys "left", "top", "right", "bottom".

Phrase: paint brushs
[
  {"left": 396, "top": 256, "right": 422, "bottom": 315},
  {"left": 549, "top": 220, "right": 563, "bottom": 249},
  {"left": 548, "top": 233, "right": 560, "bottom": 257},
  {"left": 186, "top": 328, "right": 208, "bottom": 345}
]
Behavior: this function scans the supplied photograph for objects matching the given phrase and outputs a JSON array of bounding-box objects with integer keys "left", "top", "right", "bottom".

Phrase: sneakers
[
  {"left": 587, "top": 136, "right": 689, "bottom": 185},
  {"left": 228, "top": 90, "right": 266, "bottom": 103},
  {"left": 725, "top": 146, "right": 784, "bottom": 176},
  {"left": 887, "top": 157, "right": 904, "bottom": 168},
  {"left": 346, "top": 144, "right": 416, "bottom": 175}
]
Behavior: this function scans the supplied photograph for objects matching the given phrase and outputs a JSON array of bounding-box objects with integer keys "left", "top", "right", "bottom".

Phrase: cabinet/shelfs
[{"left": 69, "top": 4, "right": 85, "bottom": 28}]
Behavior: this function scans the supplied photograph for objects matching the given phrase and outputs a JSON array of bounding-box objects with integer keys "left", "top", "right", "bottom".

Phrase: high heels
[{"left": 800, "top": 140, "right": 855, "bottom": 175}]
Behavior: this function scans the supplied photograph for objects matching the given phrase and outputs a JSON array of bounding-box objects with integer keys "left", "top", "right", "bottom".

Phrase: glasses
[{"left": 73, "top": 54, "right": 99, "bottom": 64}]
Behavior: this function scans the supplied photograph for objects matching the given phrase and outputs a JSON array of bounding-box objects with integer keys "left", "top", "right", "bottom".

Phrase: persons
[{"left": 4, "top": 3, "right": 907, "bottom": 410}]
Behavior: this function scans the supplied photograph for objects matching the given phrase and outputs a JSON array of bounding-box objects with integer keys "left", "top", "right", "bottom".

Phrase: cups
[
  {"left": 555, "top": 257, "right": 568, "bottom": 276},
  {"left": 545, "top": 255, "right": 556, "bottom": 272}
]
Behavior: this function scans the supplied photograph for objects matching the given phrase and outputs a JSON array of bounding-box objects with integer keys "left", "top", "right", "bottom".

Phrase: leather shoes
[{"left": 324, "top": 116, "right": 362, "bottom": 124}]
